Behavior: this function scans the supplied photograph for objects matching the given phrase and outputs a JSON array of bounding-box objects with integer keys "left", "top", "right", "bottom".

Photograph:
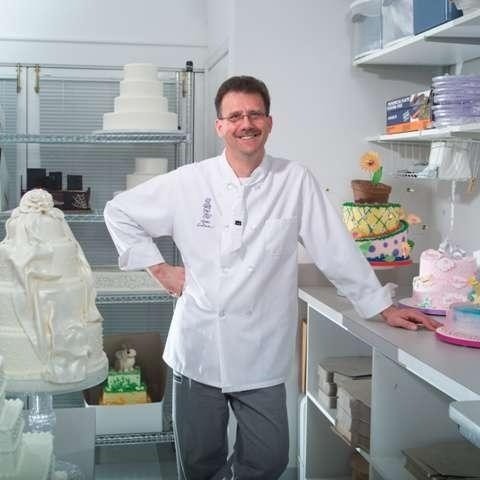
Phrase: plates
[{"left": 430, "top": 73, "right": 480, "bottom": 127}]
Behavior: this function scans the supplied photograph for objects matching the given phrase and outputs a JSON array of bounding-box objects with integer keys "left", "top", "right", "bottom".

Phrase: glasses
[{"left": 219, "top": 111, "right": 266, "bottom": 122}]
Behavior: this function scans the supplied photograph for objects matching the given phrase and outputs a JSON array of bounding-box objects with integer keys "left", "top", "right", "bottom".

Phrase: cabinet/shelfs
[
  {"left": 296, "top": 264, "right": 479, "bottom": 477},
  {"left": 346, "top": 0, "right": 479, "bottom": 143},
  {"left": 0, "top": 61, "right": 198, "bottom": 449}
]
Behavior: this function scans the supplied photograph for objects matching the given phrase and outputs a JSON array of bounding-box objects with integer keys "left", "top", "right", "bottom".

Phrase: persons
[{"left": 100, "top": 74, "right": 448, "bottom": 479}]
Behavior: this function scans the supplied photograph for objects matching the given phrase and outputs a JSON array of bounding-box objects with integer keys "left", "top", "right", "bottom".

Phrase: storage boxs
[{"left": 78, "top": 329, "right": 168, "bottom": 436}]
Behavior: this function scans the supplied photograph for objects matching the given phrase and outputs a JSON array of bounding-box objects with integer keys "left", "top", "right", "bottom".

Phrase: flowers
[{"left": 356, "top": 149, "right": 388, "bottom": 188}]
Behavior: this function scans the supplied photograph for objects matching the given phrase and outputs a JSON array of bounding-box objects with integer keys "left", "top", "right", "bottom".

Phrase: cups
[
  {"left": 26, "top": 167, "right": 46, "bottom": 191},
  {"left": 58, "top": 188, "right": 87, "bottom": 210},
  {"left": 46, "top": 169, "right": 62, "bottom": 196},
  {"left": 66, "top": 173, "right": 83, "bottom": 193}
]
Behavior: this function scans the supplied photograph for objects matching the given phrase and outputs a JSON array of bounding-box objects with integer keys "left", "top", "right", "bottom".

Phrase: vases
[{"left": 348, "top": 178, "right": 392, "bottom": 205}]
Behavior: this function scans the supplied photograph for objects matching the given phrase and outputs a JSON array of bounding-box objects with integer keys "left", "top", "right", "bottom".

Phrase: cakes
[
  {"left": 435, "top": 266, "right": 480, "bottom": 348},
  {"left": 21, "top": 167, "right": 91, "bottom": 210},
  {"left": 126, "top": 157, "right": 168, "bottom": 190},
  {"left": 398, "top": 241, "right": 480, "bottom": 315},
  {"left": 103, "top": 63, "right": 178, "bottom": 131},
  {"left": 342, "top": 151, "right": 415, "bottom": 269},
  {"left": 0, "top": 188, "right": 109, "bottom": 396},
  {"left": 99, "top": 349, "right": 152, "bottom": 404},
  {"left": 0, "top": 390, "right": 86, "bottom": 480}
]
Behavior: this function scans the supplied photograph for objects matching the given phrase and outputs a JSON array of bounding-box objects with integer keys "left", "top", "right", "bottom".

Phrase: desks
[{"left": 56, "top": 458, "right": 85, "bottom": 480}]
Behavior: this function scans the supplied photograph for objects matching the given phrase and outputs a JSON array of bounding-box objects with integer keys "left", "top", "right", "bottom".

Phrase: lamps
[{"left": 418, "top": 137, "right": 480, "bottom": 261}]
